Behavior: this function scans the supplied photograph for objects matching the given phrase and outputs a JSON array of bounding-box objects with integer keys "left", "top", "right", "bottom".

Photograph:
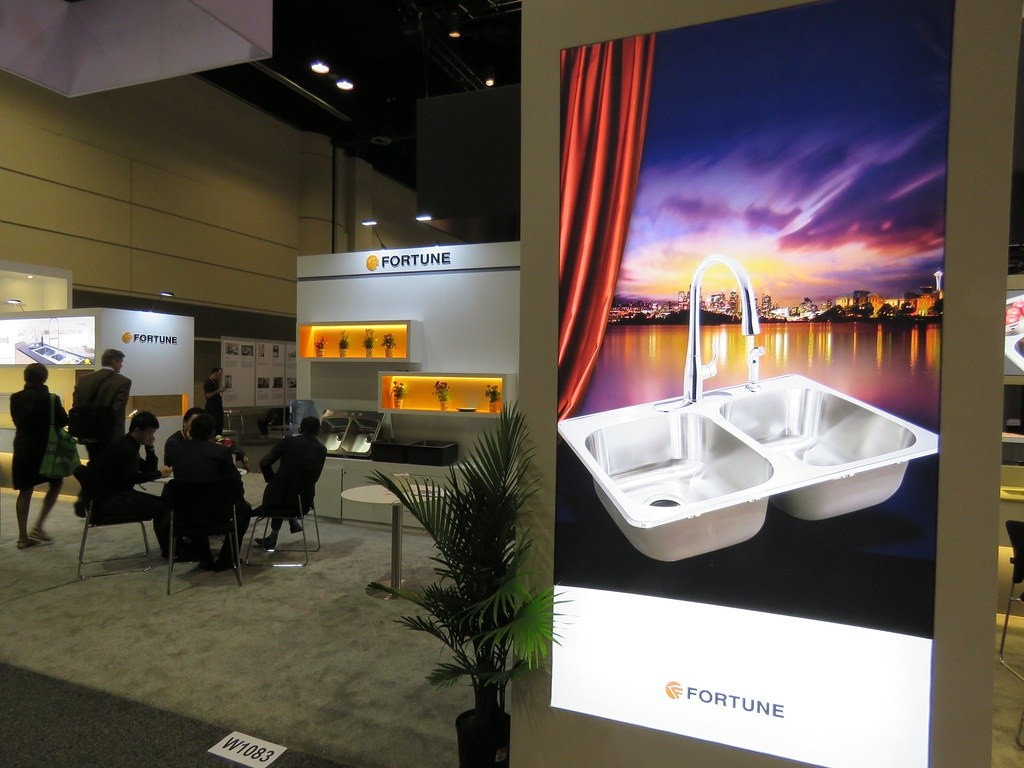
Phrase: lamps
[
  {"left": 149, "top": 292, "right": 174, "bottom": 312},
  {"left": 6, "top": 299, "right": 24, "bottom": 312},
  {"left": 417, "top": 214, "right": 440, "bottom": 247},
  {"left": 361, "top": 220, "right": 387, "bottom": 249}
]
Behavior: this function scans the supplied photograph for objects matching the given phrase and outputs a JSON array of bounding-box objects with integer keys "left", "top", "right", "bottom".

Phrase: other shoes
[
  {"left": 289, "top": 521, "right": 302, "bottom": 533},
  {"left": 29, "top": 528, "right": 50, "bottom": 541},
  {"left": 214, "top": 558, "right": 243, "bottom": 571},
  {"left": 198, "top": 554, "right": 214, "bottom": 570},
  {"left": 17, "top": 538, "right": 40, "bottom": 549},
  {"left": 255, "top": 536, "right": 276, "bottom": 550}
]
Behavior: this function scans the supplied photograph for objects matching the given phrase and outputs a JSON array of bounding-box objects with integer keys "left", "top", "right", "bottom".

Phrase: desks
[
  {"left": 341, "top": 484, "right": 449, "bottom": 600},
  {"left": 150, "top": 468, "right": 248, "bottom": 561}
]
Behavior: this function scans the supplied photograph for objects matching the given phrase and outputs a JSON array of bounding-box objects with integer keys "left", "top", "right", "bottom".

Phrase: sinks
[
  {"left": 317, "top": 416, "right": 350, "bottom": 451},
  {"left": 343, "top": 417, "right": 381, "bottom": 454},
  {"left": 708, "top": 377, "right": 918, "bottom": 519},
  {"left": 51, "top": 354, "right": 66, "bottom": 361},
  {"left": 36, "top": 348, "right": 54, "bottom": 357},
  {"left": 582, "top": 407, "right": 780, "bottom": 562}
]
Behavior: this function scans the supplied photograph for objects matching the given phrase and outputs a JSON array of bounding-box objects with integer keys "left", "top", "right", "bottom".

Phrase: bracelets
[{"left": 243, "top": 458, "right": 248, "bottom": 465}]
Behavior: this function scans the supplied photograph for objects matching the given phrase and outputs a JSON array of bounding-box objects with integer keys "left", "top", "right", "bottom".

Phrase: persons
[
  {"left": 9, "top": 363, "right": 68, "bottom": 550},
  {"left": 68, "top": 349, "right": 132, "bottom": 518},
  {"left": 87, "top": 410, "right": 188, "bottom": 559},
  {"left": 204, "top": 368, "right": 225, "bottom": 436},
  {"left": 255, "top": 416, "right": 327, "bottom": 550},
  {"left": 164, "top": 408, "right": 253, "bottom": 572}
]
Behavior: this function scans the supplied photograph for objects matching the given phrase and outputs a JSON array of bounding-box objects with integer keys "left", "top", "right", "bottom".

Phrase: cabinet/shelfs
[{"left": 308, "top": 460, "right": 470, "bottom": 532}]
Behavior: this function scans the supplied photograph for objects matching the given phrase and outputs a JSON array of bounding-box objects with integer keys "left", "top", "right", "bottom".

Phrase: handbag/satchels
[
  {"left": 38, "top": 393, "right": 82, "bottom": 478},
  {"left": 67, "top": 405, "right": 113, "bottom": 438}
]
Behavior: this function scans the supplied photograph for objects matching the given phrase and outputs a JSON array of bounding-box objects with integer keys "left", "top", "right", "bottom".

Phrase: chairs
[
  {"left": 998, "top": 521, "right": 1024, "bottom": 681},
  {"left": 74, "top": 465, "right": 151, "bottom": 576},
  {"left": 167, "top": 468, "right": 242, "bottom": 596},
  {"left": 245, "top": 460, "right": 322, "bottom": 568}
]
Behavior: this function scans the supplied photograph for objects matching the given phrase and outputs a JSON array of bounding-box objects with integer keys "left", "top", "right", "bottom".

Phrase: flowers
[
  {"left": 339, "top": 331, "right": 351, "bottom": 349},
  {"left": 391, "top": 381, "right": 407, "bottom": 400},
  {"left": 314, "top": 336, "right": 328, "bottom": 348},
  {"left": 432, "top": 381, "right": 449, "bottom": 401},
  {"left": 380, "top": 333, "right": 397, "bottom": 349},
  {"left": 485, "top": 384, "right": 501, "bottom": 402},
  {"left": 363, "top": 327, "right": 378, "bottom": 349}
]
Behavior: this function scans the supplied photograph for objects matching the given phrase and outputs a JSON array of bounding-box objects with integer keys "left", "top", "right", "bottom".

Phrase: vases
[
  {"left": 385, "top": 349, "right": 394, "bottom": 358},
  {"left": 339, "top": 348, "right": 347, "bottom": 359},
  {"left": 394, "top": 399, "right": 403, "bottom": 408},
  {"left": 316, "top": 349, "right": 325, "bottom": 358},
  {"left": 489, "top": 403, "right": 500, "bottom": 413},
  {"left": 440, "top": 401, "right": 448, "bottom": 411},
  {"left": 365, "top": 348, "right": 374, "bottom": 358}
]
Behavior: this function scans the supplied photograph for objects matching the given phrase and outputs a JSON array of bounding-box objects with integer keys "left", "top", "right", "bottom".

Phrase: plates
[{"left": 457, "top": 408, "right": 477, "bottom": 411}]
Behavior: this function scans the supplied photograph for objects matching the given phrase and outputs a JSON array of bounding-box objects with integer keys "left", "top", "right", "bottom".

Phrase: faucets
[{"left": 683, "top": 254, "right": 761, "bottom": 400}]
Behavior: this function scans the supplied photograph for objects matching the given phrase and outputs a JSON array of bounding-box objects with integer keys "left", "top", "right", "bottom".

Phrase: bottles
[
  {"left": 291, "top": 400, "right": 313, "bottom": 434},
  {"left": 128, "top": 410, "right": 138, "bottom": 420}
]
